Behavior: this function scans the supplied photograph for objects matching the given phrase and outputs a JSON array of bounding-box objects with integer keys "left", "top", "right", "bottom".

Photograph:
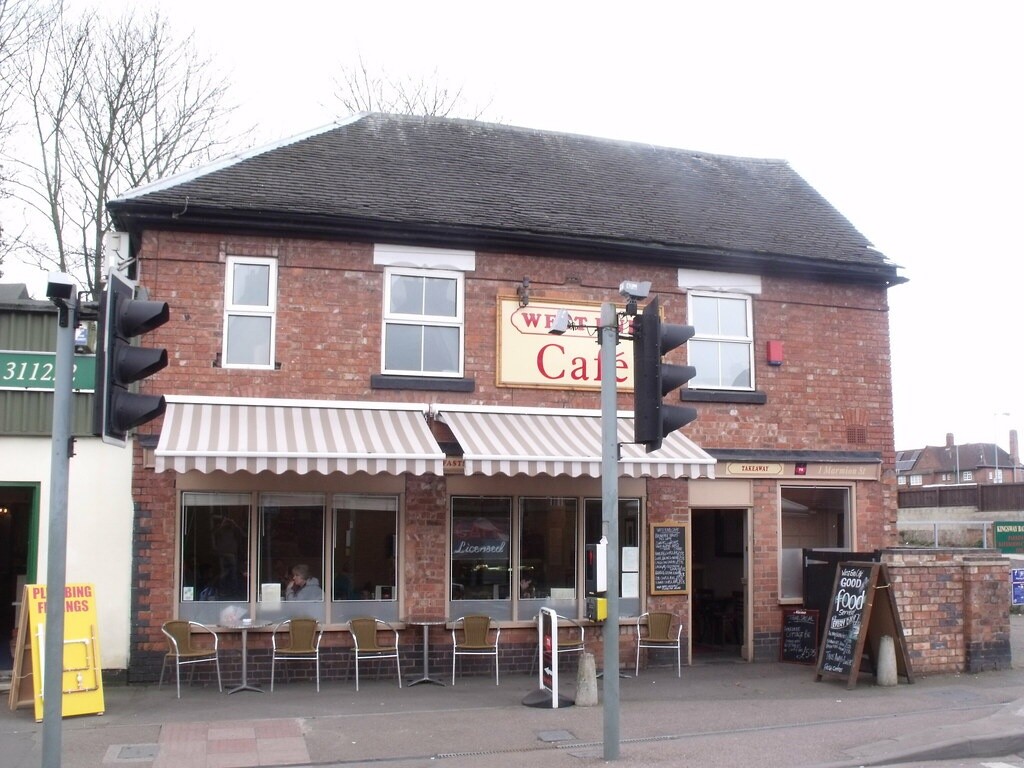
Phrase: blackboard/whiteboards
[
  {"left": 649, "top": 521, "right": 691, "bottom": 595},
  {"left": 817, "top": 561, "right": 880, "bottom": 681},
  {"left": 777, "top": 608, "right": 820, "bottom": 665}
]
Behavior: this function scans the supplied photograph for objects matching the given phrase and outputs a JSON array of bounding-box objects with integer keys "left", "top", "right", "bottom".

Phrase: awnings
[{"left": 153, "top": 394, "right": 717, "bottom": 481}]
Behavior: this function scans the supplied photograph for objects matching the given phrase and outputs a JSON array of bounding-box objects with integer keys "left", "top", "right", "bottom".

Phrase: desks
[
  {"left": 590, "top": 619, "right": 635, "bottom": 678},
  {"left": 403, "top": 621, "right": 446, "bottom": 687},
  {"left": 216, "top": 619, "right": 273, "bottom": 695}
]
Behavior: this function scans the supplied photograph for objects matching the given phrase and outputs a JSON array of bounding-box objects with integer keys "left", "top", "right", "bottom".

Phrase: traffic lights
[
  {"left": 633, "top": 295, "right": 699, "bottom": 452},
  {"left": 95, "top": 269, "right": 172, "bottom": 448}
]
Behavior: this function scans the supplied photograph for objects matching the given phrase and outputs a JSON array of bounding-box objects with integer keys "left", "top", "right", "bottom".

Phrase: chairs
[
  {"left": 635, "top": 611, "right": 683, "bottom": 678},
  {"left": 529, "top": 614, "right": 585, "bottom": 679},
  {"left": 345, "top": 617, "right": 402, "bottom": 692},
  {"left": 158, "top": 620, "right": 223, "bottom": 699},
  {"left": 697, "top": 588, "right": 739, "bottom": 648},
  {"left": 452, "top": 615, "right": 501, "bottom": 686},
  {"left": 270, "top": 618, "right": 323, "bottom": 693}
]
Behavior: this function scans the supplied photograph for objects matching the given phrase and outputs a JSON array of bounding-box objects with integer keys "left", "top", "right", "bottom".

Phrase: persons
[
  {"left": 278, "top": 563, "right": 322, "bottom": 601},
  {"left": 520, "top": 574, "right": 549, "bottom": 599}
]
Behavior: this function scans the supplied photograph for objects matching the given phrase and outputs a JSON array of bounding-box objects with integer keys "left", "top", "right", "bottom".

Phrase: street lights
[{"left": 993, "top": 412, "right": 1010, "bottom": 482}]
